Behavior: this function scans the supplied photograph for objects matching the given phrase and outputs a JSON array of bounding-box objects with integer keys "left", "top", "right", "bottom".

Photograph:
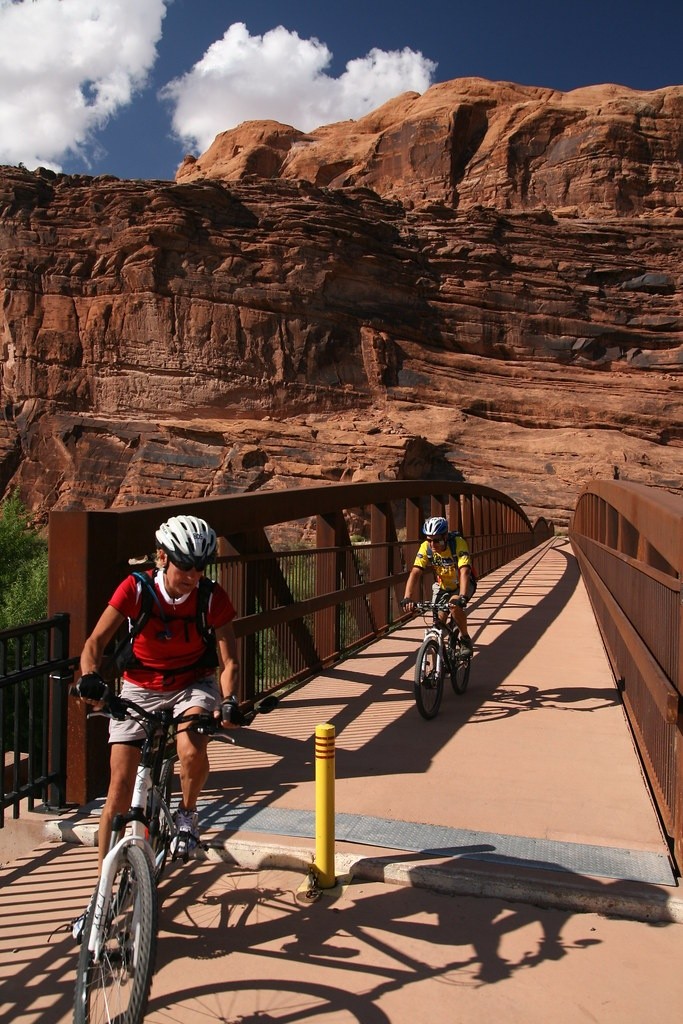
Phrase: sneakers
[
  {"left": 169, "top": 804, "right": 198, "bottom": 855},
  {"left": 72, "top": 905, "right": 93, "bottom": 938}
]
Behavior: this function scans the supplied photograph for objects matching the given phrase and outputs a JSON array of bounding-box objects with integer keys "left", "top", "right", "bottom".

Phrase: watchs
[{"left": 222, "top": 695, "right": 238, "bottom": 702}]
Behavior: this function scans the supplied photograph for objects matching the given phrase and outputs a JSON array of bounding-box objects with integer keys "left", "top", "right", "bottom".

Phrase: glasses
[
  {"left": 427, "top": 538, "right": 443, "bottom": 542},
  {"left": 169, "top": 560, "right": 208, "bottom": 572}
]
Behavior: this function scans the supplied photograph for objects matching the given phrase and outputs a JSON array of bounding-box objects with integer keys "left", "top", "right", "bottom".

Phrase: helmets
[
  {"left": 155, "top": 514, "right": 219, "bottom": 566},
  {"left": 423, "top": 517, "right": 448, "bottom": 536}
]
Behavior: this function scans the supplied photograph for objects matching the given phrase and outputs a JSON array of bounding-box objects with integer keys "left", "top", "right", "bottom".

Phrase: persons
[
  {"left": 74, "top": 515, "right": 241, "bottom": 938},
  {"left": 402, "top": 517, "right": 475, "bottom": 684}
]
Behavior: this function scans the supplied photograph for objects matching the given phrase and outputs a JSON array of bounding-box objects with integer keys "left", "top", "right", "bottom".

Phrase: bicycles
[
  {"left": 402, "top": 598, "right": 472, "bottom": 723},
  {"left": 65, "top": 685, "right": 242, "bottom": 1024}
]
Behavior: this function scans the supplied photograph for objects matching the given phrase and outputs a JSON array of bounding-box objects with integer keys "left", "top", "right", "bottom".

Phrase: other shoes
[
  {"left": 422, "top": 673, "right": 432, "bottom": 689},
  {"left": 460, "top": 637, "right": 473, "bottom": 656}
]
